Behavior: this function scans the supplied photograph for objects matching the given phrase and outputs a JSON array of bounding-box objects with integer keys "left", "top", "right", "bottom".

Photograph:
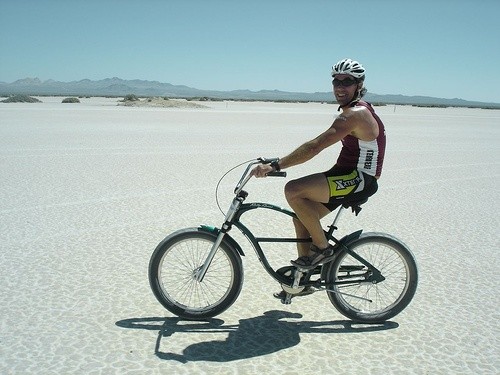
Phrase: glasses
[{"left": 332, "top": 80, "right": 359, "bottom": 87}]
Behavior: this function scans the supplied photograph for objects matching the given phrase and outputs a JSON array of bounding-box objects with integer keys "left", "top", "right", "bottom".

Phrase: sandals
[
  {"left": 273, "top": 289, "right": 312, "bottom": 299},
  {"left": 290, "top": 244, "right": 335, "bottom": 270}
]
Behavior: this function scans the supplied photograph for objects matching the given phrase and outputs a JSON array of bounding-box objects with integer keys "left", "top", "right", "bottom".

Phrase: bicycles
[{"left": 148, "top": 157, "right": 420, "bottom": 324}]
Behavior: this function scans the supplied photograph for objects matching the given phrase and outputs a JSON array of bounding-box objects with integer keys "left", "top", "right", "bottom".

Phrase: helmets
[{"left": 331, "top": 58, "right": 365, "bottom": 78}]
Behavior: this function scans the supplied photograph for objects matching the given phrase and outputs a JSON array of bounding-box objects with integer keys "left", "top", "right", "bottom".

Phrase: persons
[{"left": 249, "top": 57, "right": 386, "bottom": 301}]
